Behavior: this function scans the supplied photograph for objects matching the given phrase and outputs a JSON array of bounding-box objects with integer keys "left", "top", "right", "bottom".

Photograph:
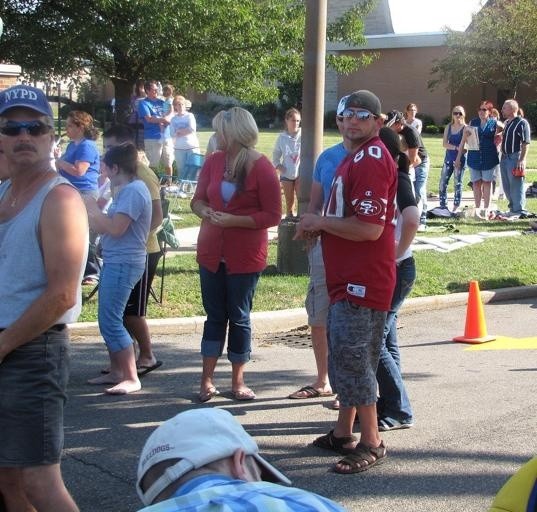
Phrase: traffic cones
[{"left": 454, "top": 279, "right": 495, "bottom": 344}]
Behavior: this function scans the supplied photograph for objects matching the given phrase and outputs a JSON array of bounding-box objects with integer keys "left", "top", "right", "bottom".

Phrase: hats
[
  {"left": 343, "top": 88, "right": 381, "bottom": 117},
  {"left": 0, "top": 85, "right": 55, "bottom": 118},
  {"left": 136, "top": 403, "right": 294, "bottom": 507},
  {"left": 334, "top": 97, "right": 351, "bottom": 112}
]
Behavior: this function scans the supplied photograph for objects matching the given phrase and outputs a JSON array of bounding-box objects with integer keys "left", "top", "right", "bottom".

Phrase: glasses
[
  {"left": 0, "top": 120, "right": 54, "bottom": 135},
  {"left": 476, "top": 108, "right": 487, "bottom": 112},
  {"left": 452, "top": 112, "right": 463, "bottom": 116},
  {"left": 337, "top": 113, "right": 342, "bottom": 121},
  {"left": 343, "top": 111, "right": 381, "bottom": 120}
]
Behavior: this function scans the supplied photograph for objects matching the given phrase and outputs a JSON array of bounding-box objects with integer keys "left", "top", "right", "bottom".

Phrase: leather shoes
[{"left": 287, "top": 384, "right": 333, "bottom": 400}]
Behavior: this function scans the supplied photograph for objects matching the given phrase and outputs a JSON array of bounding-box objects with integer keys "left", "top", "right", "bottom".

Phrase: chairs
[{"left": 160, "top": 150, "right": 203, "bottom": 213}]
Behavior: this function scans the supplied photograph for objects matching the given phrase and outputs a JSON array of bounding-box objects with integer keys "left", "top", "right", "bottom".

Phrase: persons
[
  {"left": 288, "top": 90, "right": 428, "bottom": 473},
  {"left": 53, "top": 81, "right": 201, "bottom": 396},
  {"left": 436, "top": 99, "right": 530, "bottom": 220},
  {"left": 274, "top": 108, "right": 303, "bottom": 217},
  {"left": 133, "top": 407, "right": 350, "bottom": 511},
  {"left": 0, "top": 88, "right": 84, "bottom": 512},
  {"left": 190, "top": 107, "right": 282, "bottom": 401}
]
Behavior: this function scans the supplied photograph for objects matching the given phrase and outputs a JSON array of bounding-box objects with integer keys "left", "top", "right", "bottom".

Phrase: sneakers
[
  {"left": 177, "top": 191, "right": 186, "bottom": 199},
  {"left": 375, "top": 413, "right": 415, "bottom": 431},
  {"left": 165, "top": 185, "right": 179, "bottom": 193}
]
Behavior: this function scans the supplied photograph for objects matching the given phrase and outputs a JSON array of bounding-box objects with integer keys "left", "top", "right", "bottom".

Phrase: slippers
[
  {"left": 101, "top": 365, "right": 112, "bottom": 374},
  {"left": 197, "top": 385, "right": 220, "bottom": 404},
  {"left": 331, "top": 394, "right": 338, "bottom": 410},
  {"left": 134, "top": 355, "right": 163, "bottom": 377},
  {"left": 229, "top": 383, "right": 257, "bottom": 402}
]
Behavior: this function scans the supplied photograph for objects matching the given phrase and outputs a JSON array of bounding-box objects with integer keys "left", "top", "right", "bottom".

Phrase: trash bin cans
[{"left": 277, "top": 216, "right": 311, "bottom": 274}]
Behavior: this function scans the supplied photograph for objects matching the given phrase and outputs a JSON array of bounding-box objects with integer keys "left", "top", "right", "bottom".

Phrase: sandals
[
  {"left": 335, "top": 438, "right": 388, "bottom": 477},
  {"left": 311, "top": 426, "right": 358, "bottom": 454}
]
